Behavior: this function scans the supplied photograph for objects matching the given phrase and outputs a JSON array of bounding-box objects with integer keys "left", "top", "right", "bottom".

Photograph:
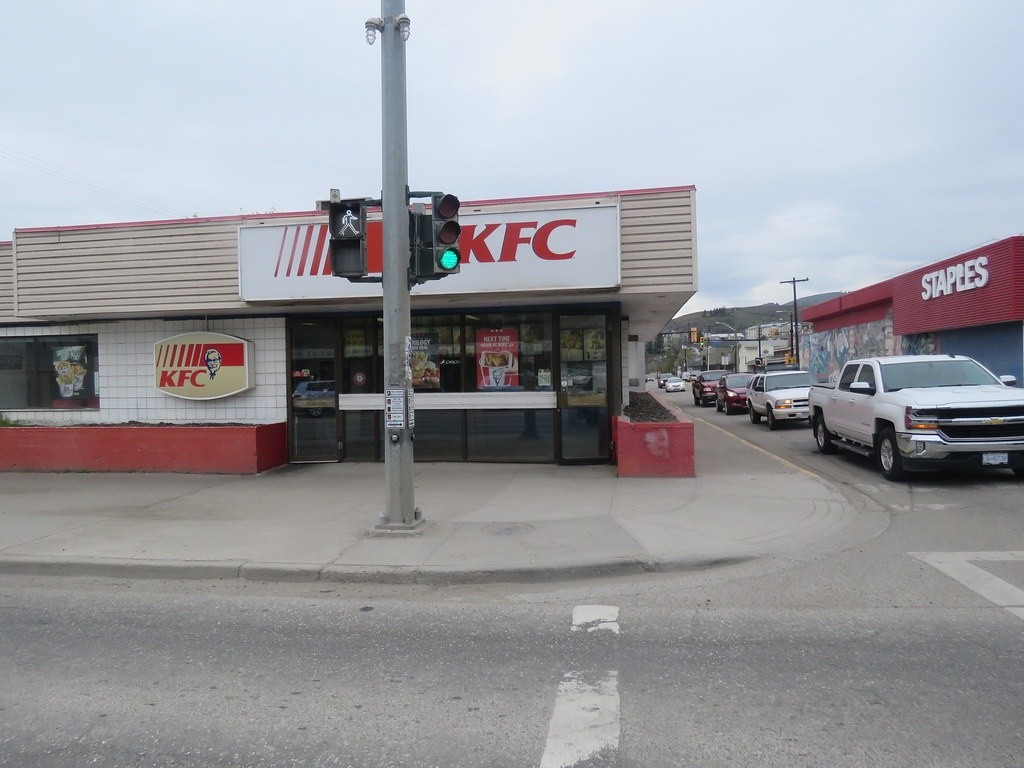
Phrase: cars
[
  {"left": 682, "top": 370, "right": 700, "bottom": 383},
  {"left": 664, "top": 377, "right": 686, "bottom": 393},
  {"left": 645, "top": 374, "right": 655, "bottom": 383},
  {"left": 715, "top": 372, "right": 757, "bottom": 415},
  {"left": 570, "top": 363, "right": 593, "bottom": 389}
]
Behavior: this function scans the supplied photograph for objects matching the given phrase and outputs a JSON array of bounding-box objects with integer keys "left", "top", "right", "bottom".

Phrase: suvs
[
  {"left": 692, "top": 369, "right": 730, "bottom": 408},
  {"left": 746, "top": 370, "right": 817, "bottom": 431},
  {"left": 658, "top": 374, "right": 674, "bottom": 388}
]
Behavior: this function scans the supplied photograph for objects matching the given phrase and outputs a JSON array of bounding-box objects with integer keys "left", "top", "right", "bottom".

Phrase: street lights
[
  {"left": 715, "top": 322, "right": 739, "bottom": 374},
  {"left": 776, "top": 310, "right": 793, "bottom": 358}
]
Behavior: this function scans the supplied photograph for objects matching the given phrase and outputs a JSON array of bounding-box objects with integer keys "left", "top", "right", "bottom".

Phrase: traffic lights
[
  {"left": 784, "top": 353, "right": 789, "bottom": 364},
  {"left": 432, "top": 193, "right": 461, "bottom": 274},
  {"left": 770, "top": 327, "right": 776, "bottom": 338},
  {"left": 326, "top": 203, "right": 368, "bottom": 277},
  {"left": 700, "top": 337, "right": 705, "bottom": 350}
]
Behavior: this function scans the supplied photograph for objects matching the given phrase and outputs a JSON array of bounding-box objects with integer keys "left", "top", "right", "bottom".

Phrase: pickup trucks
[{"left": 808, "top": 352, "right": 1024, "bottom": 478}]
